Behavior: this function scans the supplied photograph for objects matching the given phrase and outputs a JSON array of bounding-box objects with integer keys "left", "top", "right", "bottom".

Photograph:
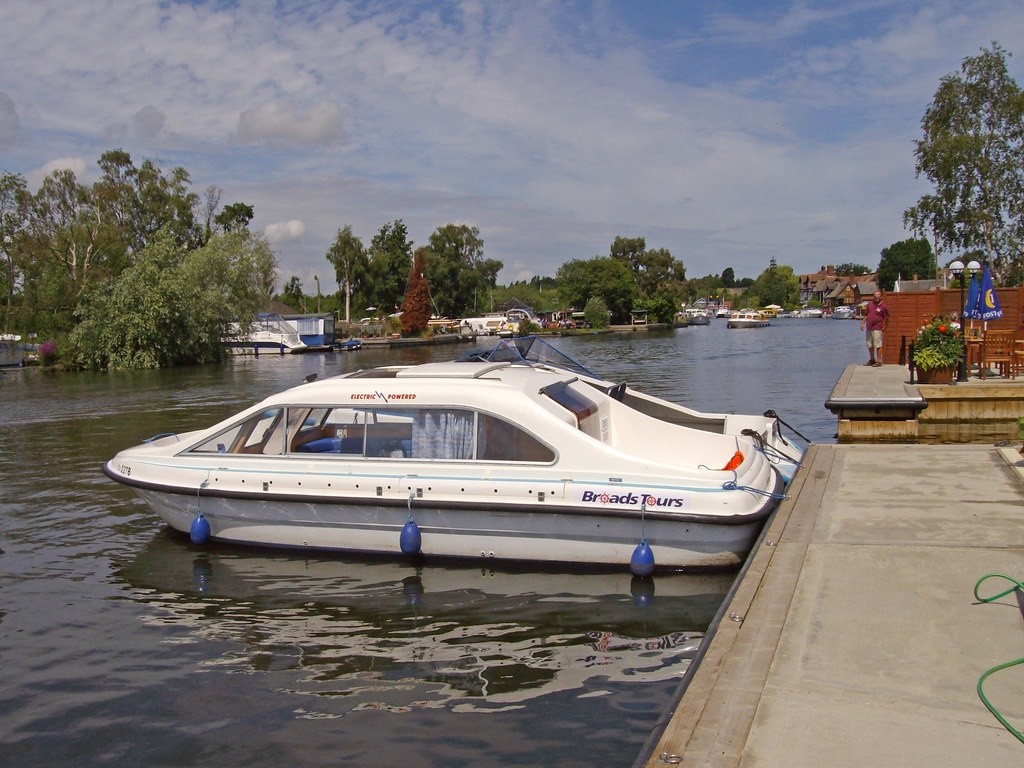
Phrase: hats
[{"left": 874, "top": 290, "right": 881, "bottom": 297}]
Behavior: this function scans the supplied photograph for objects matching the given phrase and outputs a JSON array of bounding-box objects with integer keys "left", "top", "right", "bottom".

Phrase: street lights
[{"left": 949, "top": 258, "right": 985, "bottom": 382}]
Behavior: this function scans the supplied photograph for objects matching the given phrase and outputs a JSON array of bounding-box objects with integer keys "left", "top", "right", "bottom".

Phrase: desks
[{"left": 967, "top": 338, "right": 1024, "bottom": 378}]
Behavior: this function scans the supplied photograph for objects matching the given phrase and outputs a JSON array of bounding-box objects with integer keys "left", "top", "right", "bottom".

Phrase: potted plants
[
  {"left": 362, "top": 328, "right": 369, "bottom": 339},
  {"left": 372, "top": 328, "right": 379, "bottom": 339},
  {"left": 914, "top": 343, "right": 964, "bottom": 384},
  {"left": 389, "top": 315, "right": 401, "bottom": 339}
]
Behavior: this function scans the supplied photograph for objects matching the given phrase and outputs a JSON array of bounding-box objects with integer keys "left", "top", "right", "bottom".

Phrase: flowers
[{"left": 916, "top": 311, "right": 962, "bottom": 343}]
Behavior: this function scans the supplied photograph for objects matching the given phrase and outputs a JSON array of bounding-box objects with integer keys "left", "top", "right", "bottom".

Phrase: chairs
[
  {"left": 977, "top": 329, "right": 1016, "bottom": 380},
  {"left": 1014, "top": 350, "right": 1024, "bottom": 376}
]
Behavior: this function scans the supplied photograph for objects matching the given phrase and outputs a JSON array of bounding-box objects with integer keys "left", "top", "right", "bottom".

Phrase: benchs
[{"left": 297, "top": 437, "right": 412, "bottom": 457}]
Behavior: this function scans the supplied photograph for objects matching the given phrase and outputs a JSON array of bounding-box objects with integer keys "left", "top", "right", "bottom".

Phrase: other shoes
[
  {"left": 863, "top": 359, "right": 875, "bottom": 366},
  {"left": 873, "top": 362, "right": 882, "bottom": 366}
]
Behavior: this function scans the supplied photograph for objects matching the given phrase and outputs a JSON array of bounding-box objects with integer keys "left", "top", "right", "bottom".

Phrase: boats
[
  {"left": 117, "top": 528, "right": 740, "bottom": 650},
  {"left": 832, "top": 306, "right": 856, "bottom": 319},
  {"left": 97, "top": 332, "right": 812, "bottom": 573},
  {"left": 678, "top": 304, "right": 790, "bottom": 330},
  {"left": 386, "top": 308, "right": 546, "bottom": 340},
  {"left": 788, "top": 305, "right": 822, "bottom": 318},
  {"left": 221, "top": 313, "right": 362, "bottom": 353}
]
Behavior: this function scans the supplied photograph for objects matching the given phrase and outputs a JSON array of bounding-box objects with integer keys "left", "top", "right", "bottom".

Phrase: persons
[
  {"left": 859, "top": 290, "right": 892, "bottom": 368},
  {"left": 931, "top": 310, "right": 963, "bottom": 332}
]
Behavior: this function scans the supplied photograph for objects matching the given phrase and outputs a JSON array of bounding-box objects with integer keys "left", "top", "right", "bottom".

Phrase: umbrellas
[
  {"left": 960, "top": 276, "right": 980, "bottom": 331},
  {"left": 972, "top": 265, "right": 1004, "bottom": 332}
]
